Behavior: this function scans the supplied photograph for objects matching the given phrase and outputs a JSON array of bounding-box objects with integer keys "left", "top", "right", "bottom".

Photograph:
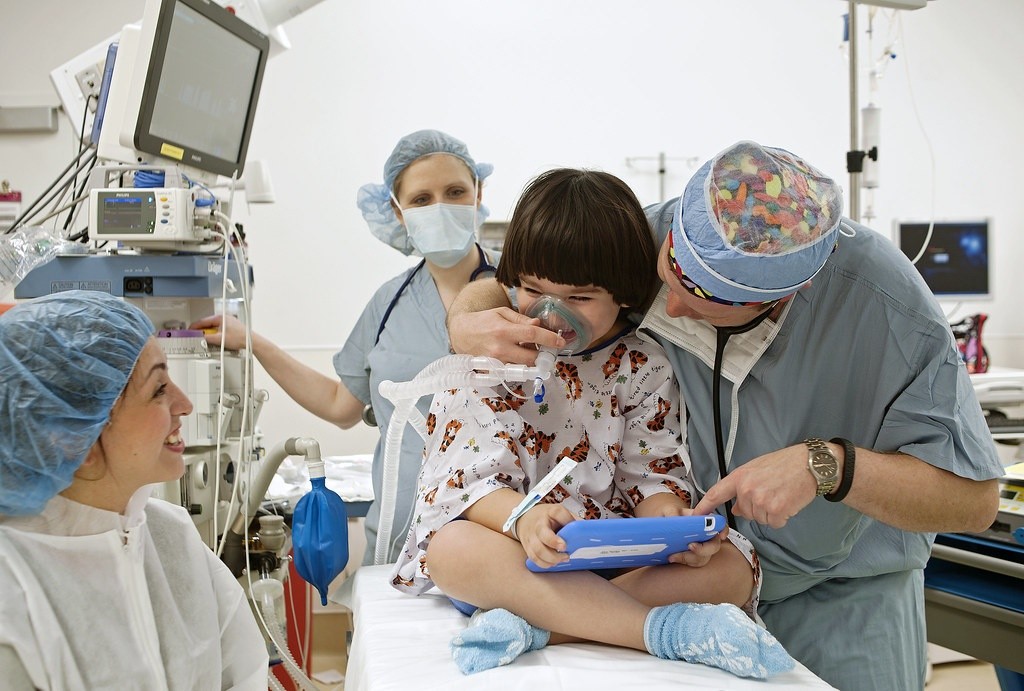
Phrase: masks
[
  {"left": 389, "top": 174, "right": 478, "bottom": 269},
  {"left": 635, "top": 292, "right": 796, "bottom": 388}
]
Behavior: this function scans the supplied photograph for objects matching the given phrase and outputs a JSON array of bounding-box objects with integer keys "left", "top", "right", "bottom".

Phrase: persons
[
  {"left": 391, "top": 169, "right": 796, "bottom": 682},
  {"left": 0, "top": 291, "right": 271, "bottom": 691},
  {"left": 446, "top": 143, "right": 1005, "bottom": 689},
  {"left": 188, "top": 128, "right": 502, "bottom": 566}
]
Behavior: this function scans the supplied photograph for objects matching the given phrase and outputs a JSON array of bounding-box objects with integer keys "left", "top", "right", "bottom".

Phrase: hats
[{"left": 666, "top": 140, "right": 857, "bottom": 308}]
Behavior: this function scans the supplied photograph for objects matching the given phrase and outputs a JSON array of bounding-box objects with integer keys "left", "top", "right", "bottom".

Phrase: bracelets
[{"left": 824, "top": 438, "right": 855, "bottom": 503}]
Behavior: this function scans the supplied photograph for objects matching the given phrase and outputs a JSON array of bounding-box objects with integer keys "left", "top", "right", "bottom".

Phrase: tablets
[{"left": 526, "top": 515, "right": 726, "bottom": 572}]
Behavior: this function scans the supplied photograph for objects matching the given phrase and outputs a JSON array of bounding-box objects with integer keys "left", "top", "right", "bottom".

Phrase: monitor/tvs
[
  {"left": 895, "top": 218, "right": 995, "bottom": 300},
  {"left": 133, "top": 0, "right": 270, "bottom": 180}
]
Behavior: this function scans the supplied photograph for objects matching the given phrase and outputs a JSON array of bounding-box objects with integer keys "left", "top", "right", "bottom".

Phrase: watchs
[{"left": 803, "top": 438, "right": 839, "bottom": 497}]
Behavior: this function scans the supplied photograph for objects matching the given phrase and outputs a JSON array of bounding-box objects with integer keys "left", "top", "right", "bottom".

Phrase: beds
[{"left": 254, "top": 444, "right": 838, "bottom": 690}]
